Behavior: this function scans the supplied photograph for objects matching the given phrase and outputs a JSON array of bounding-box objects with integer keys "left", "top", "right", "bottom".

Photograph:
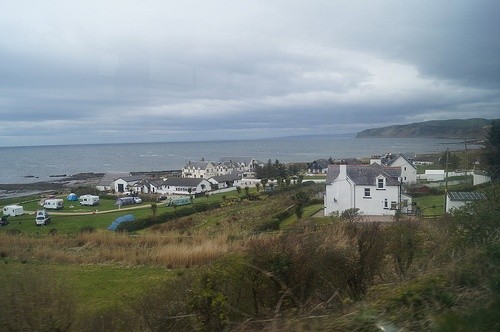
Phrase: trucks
[
  {"left": 3, "top": 206, "right": 23, "bottom": 217},
  {"left": 44, "top": 199, "right": 63, "bottom": 210},
  {"left": 116, "top": 196, "right": 142, "bottom": 206},
  {"left": 80, "top": 195, "right": 99, "bottom": 206},
  {"left": 36, "top": 210, "right": 51, "bottom": 226}
]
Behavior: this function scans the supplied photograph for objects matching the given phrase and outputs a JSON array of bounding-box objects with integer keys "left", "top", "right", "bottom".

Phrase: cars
[
  {"left": 157, "top": 195, "right": 167, "bottom": 200},
  {"left": 0, "top": 218, "right": 9, "bottom": 227}
]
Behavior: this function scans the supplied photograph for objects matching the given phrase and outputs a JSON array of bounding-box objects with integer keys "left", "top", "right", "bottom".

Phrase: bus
[{"left": 166, "top": 197, "right": 192, "bottom": 208}]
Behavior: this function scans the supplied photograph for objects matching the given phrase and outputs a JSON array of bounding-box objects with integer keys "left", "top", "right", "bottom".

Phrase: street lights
[{"left": 398, "top": 177, "right": 401, "bottom": 211}]
